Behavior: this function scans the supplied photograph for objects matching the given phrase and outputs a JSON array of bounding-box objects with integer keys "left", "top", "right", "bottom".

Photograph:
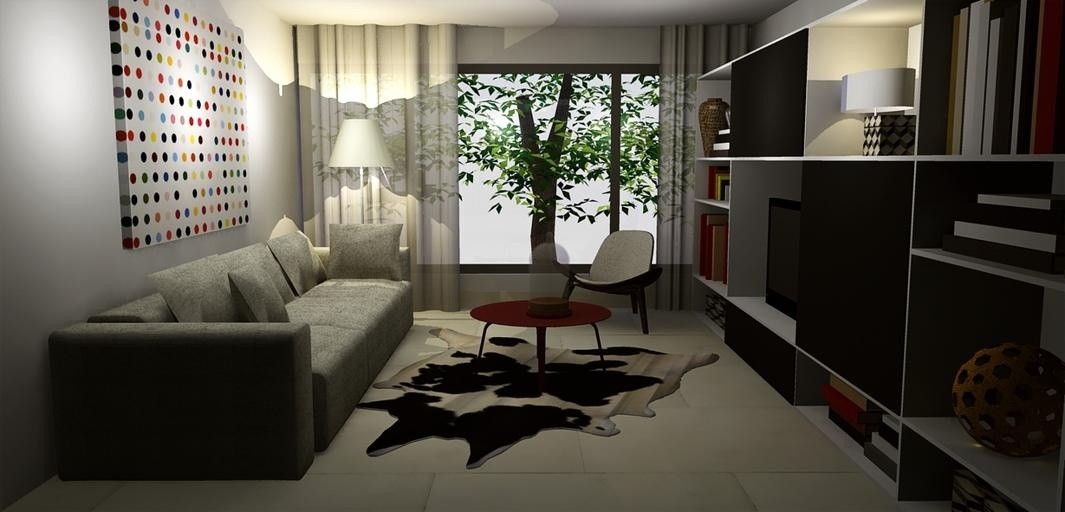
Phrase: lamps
[
  {"left": 327, "top": 117, "right": 396, "bottom": 225},
  {"left": 838, "top": 66, "right": 917, "bottom": 117}
]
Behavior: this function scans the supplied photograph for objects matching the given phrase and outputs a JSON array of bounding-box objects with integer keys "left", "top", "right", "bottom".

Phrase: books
[
  {"left": 699, "top": 213, "right": 728, "bottom": 285},
  {"left": 706, "top": 165, "right": 729, "bottom": 201}
]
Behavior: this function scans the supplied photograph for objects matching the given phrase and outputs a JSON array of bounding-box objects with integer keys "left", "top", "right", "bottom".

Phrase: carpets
[{"left": 354, "top": 327, "right": 722, "bottom": 471}]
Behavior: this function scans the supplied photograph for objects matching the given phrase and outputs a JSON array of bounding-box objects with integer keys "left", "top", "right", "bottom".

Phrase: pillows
[
  {"left": 265, "top": 229, "right": 329, "bottom": 298},
  {"left": 327, "top": 222, "right": 405, "bottom": 284},
  {"left": 142, "top": 251, "right": 259, "bottom": 323},
  {"left": 223, "top": 261, "right": 291, "bottom": 323}
]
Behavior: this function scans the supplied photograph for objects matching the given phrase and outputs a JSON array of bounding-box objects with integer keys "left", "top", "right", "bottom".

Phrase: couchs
[{"left": 47, "top": 245, "right": 416, "bottom": 485}]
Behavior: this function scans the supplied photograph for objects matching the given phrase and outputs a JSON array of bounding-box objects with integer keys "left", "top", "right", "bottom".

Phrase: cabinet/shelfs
[{"left": 689, "top": 0, "right": 1065, "bottom": 510}]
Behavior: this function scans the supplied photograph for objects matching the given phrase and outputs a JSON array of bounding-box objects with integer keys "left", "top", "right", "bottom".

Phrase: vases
[{"left": 697, "top": 96, "right": 731, "bottom": 158}]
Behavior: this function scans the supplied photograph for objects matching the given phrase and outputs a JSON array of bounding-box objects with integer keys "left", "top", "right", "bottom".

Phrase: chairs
[{"left": 552, "top": 229, "right": 665, "bottom": 335}]
